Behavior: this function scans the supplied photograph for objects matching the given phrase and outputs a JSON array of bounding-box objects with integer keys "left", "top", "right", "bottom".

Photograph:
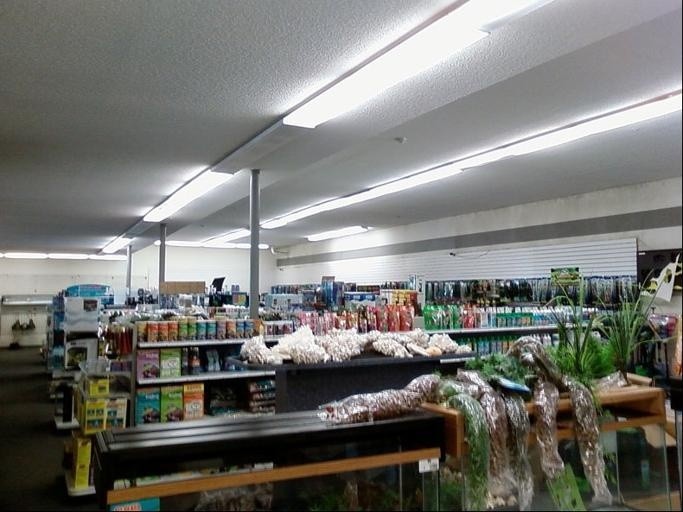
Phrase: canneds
[{"left": 135, "top": 319, "right": 255, "bottom": 343}]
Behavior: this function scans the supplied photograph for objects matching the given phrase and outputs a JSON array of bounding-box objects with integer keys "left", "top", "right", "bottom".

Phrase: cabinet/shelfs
[
  {"left": 100, "top": 297, "right": 424, "bottom": 328},
  {"left": 63, "top": 323, "right": 134, "bottom": 497},
  {"left": 124, "top": 310, "right": 423, "bottom": 429},
  {"left": 50, "top": 297, "right": 99, "bottom": 427},
  {"left": 421, "top": 300, "right": 601, "bottom": 359}
]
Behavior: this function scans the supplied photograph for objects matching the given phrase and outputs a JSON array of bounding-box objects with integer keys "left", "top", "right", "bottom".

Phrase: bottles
[
  {"left": 181, "top": 347, "right": 200, "bottom": 369},
  {"left": 208, "top": 285, "right": 229, "bottom": 305}
]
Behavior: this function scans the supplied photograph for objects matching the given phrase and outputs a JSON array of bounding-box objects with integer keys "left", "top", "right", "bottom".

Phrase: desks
[{"left": 422, "top": 373, "right": 683, "bottom": 512}]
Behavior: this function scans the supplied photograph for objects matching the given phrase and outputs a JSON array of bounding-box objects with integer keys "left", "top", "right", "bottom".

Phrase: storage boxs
[{"left": 641, "top": 415, "right": 681, "bottom": 486}]
